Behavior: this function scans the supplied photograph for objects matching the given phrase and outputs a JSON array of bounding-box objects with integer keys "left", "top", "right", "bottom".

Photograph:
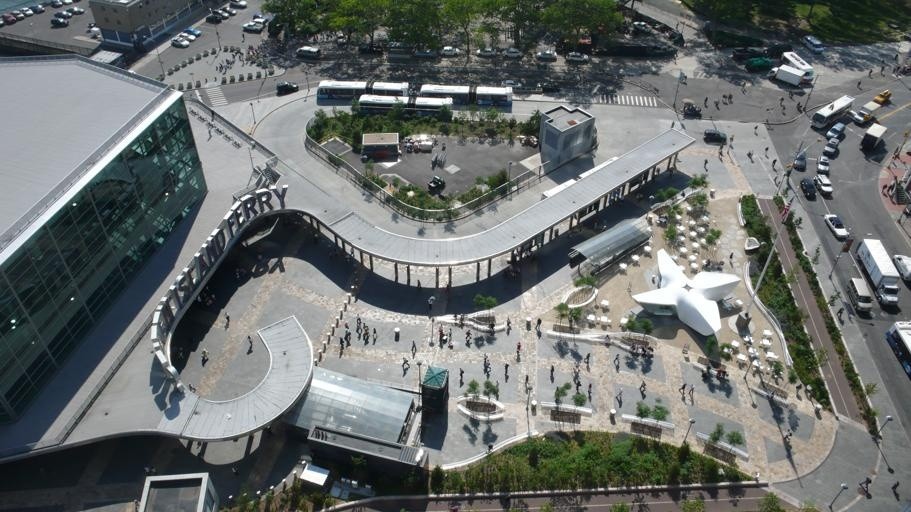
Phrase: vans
[
  {"left": 802, "top": 36, "right": 824, "bottom": 54},
  {"left": 847, "top": 277, "right": 873, "bottom": 313},
  {"left": 296, "top": 46, "right": 320, "bottom": 58}
]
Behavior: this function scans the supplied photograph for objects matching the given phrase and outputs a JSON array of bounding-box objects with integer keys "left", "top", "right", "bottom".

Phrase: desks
[
  {"left": 723, "top": 328, "right": 781, "bottom": 377},
  {"left": 615, "top": 197, "right": 711, "bottom": 278},
  {"left": 586, "top": 296, "right": 628, "bottom": 329}
]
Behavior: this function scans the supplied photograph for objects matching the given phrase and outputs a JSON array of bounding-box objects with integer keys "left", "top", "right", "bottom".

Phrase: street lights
[
  {"left": 417, "top": 360, "right": 424, "bottom": 408},
  {"left": 743, "top": 353, "right": 757, "bottom": 379},
  {"left": 142, "top": 24, "right": 167, "bottom": 78},
  {"left": 526, "top": 385, "right": 534, "bottom": 410},
  {"left": 209, "top": 7, "right": 222, "bottom": 52},
  {"left": 250, "top": 102, "right": 257, "bottom": 126},
  {"left": 775, "top": 174, "right": 790, "bottom": 196},
  {"left": 829, "top": 483, "right": 848, "bottom": 508},
  {"left": 681, "top": 418, "right": 697, "bottom": 446},
  {"left": 507, "top": 160, "right": 514, "bottom": 192},
  {"left": 429, "top": 295, "right": 436, "bottom": 319},
  {"left": 875, "top": 414, "right": 894, "bottom": 438}
]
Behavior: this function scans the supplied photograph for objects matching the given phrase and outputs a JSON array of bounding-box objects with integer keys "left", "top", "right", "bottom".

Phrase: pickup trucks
[{"left": 429, "top": 176, "right": 445, "bottom": 190}]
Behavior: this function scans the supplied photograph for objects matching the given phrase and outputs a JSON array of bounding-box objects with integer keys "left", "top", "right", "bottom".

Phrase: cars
[
  {"left": 794, "top": 89, "right": 893, "bottom": 200},
  {"left": 206, "top": 0, "right": 248, "bottom": 24},
  {"left": 824, "top": 213, "right": 850, "bottom": 239},
  {"left": 336, "top": 32, "right": 591, "bottom": 62},
  {"left": 501, "top": 80, "right": 523, "bottom": 89},
  {"left": 171, "top": 26, "right": 203, "bottom": 49},
  {"left": 0, "top": 0, "right": 85, "bottom": 28},
  {"left": 681, "top": 104, "right": 703, "bottom": 115},
  {"left": 893, "top": 253, "right": 911, "bottom": 282},
  {"left": 404, "top": 134, "right": 438, "bottom": 152},
  {"left": 242, "top": 13, "right": 272, "bottom": 33},
  {"left": 527, "top": 135, "right": 540, "bottom": 149},
  {"left": 633, "top": 21, "right": 685, "bottom": 49}
]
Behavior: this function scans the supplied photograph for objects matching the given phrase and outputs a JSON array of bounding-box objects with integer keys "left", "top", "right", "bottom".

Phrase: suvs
[
  {"left": 704, "top": 128, "right": 728, "bottom": 143},
  {"left": 276, "top": 81, "right": 300, "bottom": 94},
  {"left": 538, "top": 78, "right": 561, "bottom": 94}
]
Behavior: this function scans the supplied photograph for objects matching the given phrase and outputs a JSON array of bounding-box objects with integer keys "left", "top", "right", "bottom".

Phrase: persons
[
  {"left": 835, "top": 304, "right": 846, "bottom": 321},
  {"left": 210, "top": 108, "right": 215, "bottom": 123},
  {"left": 783, "top": 428, "right": 793, "bottom": 445},
  {"left": 891, "top": 481, "right": 901, "bottom": 492},
  {"left": 214, "top": 47, "right": 258, "bottom": 74},
  {"left": 858, "top": 475, "right": 872, "bottom": 493},
  {"left": 669, "top": 52, "right": 909, "bottom": 197},
  {"left": 241, "top": 32, "right": 245, "bottom": 42}
]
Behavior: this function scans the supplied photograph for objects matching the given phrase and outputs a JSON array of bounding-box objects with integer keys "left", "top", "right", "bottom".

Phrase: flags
[{"left": 780, "top": 198, "right": 796, "bottom": 223}]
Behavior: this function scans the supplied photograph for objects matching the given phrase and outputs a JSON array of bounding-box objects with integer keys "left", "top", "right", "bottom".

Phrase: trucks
[
  {"left": 734, "top": 42, "right": 816, "bottom": 88},
  {"left": 856, "top": 238, "right": 900, "bottom": 306}
]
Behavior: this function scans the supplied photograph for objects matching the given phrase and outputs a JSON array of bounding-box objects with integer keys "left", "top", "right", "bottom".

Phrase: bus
[
  {"left": 317, "top": 80, "right": 514, "bottom": 118},
  {"left": 887, "top": 319, "right": 911, "bottom": 379}
]
